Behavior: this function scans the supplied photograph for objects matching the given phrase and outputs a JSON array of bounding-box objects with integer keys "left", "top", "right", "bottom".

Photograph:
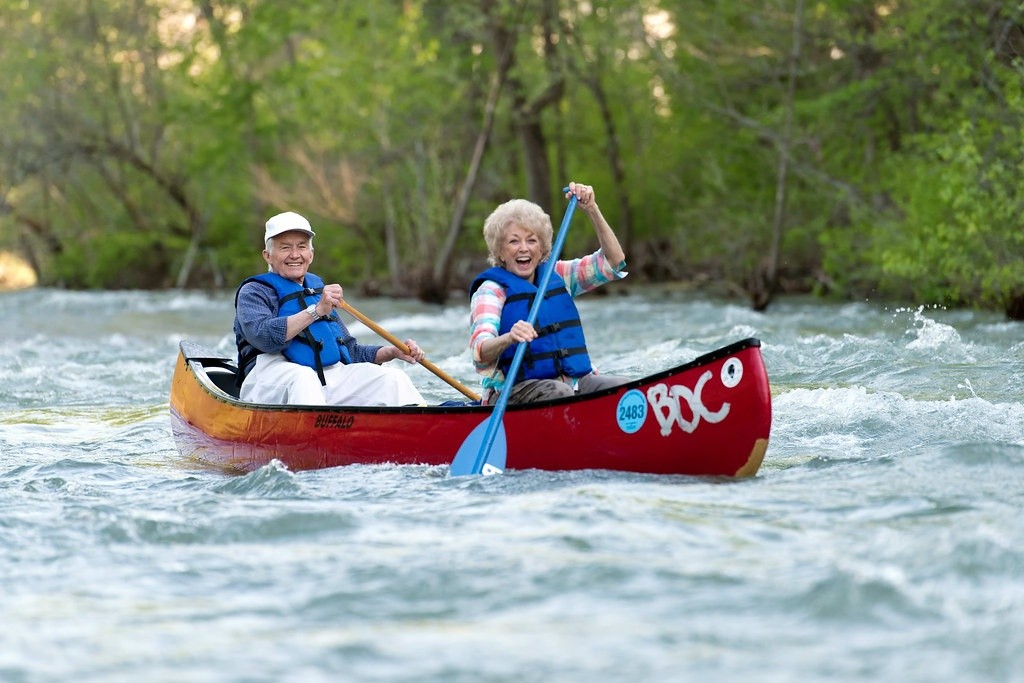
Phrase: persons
[
  {"left": 468, "top": 181, "right": 634, "bottom": 406},
  {"left": 232, "top": 211, "right": 427, "bottom": 407}
]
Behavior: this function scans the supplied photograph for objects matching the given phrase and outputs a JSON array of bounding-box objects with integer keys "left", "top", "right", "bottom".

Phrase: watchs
[{"left": 306, "top": 304, "right": 320, "bottom": 321}]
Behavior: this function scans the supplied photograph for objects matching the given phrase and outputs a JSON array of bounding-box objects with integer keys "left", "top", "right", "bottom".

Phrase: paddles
[
  {"left": 446, "top": 185, "right": 584, "bottom": 481},
  {"left": 338, "top": 299, "right": 484, "bottom": 404}
]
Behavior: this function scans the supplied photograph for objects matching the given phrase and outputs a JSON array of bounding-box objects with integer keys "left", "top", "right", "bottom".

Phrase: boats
[{"left": 170, "top": 184, "right": 773, "bottom": 478}]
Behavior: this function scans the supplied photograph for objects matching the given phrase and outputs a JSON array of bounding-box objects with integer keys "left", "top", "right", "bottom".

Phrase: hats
[{"left": 264, "top": 211, "right": 315, "bottom": 245}]
